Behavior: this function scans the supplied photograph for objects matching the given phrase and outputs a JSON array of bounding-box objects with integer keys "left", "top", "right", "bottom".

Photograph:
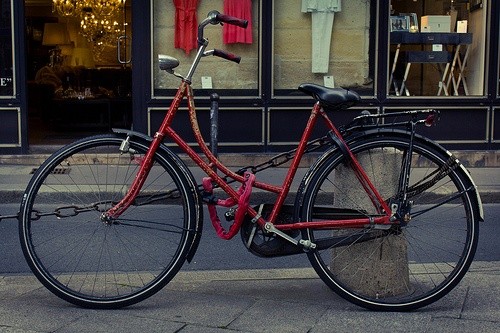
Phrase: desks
[
  {"left": 49, "top": 96, "right": 129, "bottom": 133},
  {"left": 389, "top": 30, "right": 473, "bottom": 97}
]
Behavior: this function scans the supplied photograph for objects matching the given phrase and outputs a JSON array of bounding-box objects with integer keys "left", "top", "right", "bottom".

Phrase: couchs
[{"left": 34, "top": 64, "right": 133, "bottom": 124}]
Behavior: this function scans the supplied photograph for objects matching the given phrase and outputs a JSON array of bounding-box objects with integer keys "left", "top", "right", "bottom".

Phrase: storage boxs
[{"left": 420, "top": 15, "right": 451, "bottom": 33}]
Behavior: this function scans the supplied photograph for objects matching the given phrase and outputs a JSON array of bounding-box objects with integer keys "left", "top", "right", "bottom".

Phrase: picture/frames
[
  {"left": 389, "top": 16, "right": 410, "bottom": 33},
  {"left": 398, "top": 12, "right": 419, "bottom": 34}
]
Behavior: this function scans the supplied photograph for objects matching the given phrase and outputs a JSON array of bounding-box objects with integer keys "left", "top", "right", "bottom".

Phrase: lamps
[{"left": 41, "top": 22, "right": 72, "bottom": 67}]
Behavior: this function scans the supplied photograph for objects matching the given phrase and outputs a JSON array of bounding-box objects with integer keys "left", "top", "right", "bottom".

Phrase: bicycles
[{"left": 18, "top": 11, "right": 485, "bottom": 313}]
